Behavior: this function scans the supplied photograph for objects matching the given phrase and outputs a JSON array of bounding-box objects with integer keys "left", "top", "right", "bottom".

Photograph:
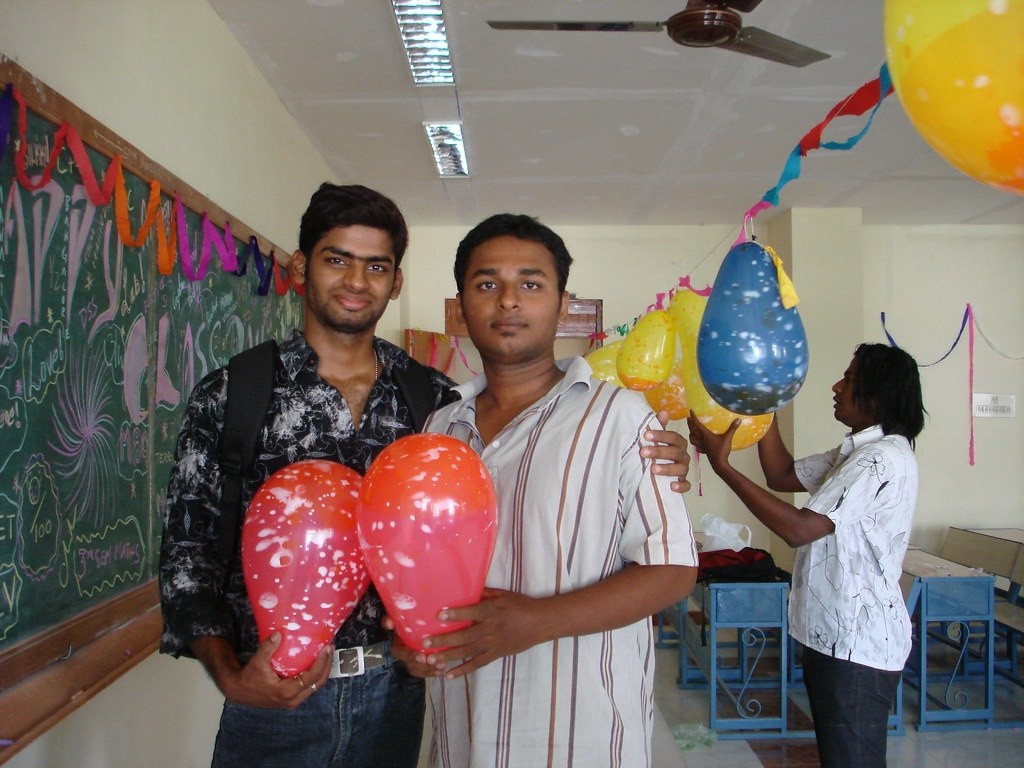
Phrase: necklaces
[{"left": 371, "top": 343, "right": 383, "bottom": 389}]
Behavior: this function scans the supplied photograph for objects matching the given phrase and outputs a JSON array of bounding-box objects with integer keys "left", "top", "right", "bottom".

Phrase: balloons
[
  {"left": 357, "top": 428, "right": 500, "bottom": 658},
  {"left": 883, "top": 0, "right": 1024, "bottom": 204},
  {"left": 578, "top": 236, "right": 814, "bottom": 453},
  {"left": 239, "top": 457, "right": 374, "bottom": 680}
]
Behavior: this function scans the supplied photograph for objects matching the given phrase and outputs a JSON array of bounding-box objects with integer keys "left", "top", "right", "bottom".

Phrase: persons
[
  {"left": 416, "top": 209, "right": 707, "bottom": 767},
  {"left": 156, "top": 181, "right": 694, "bottom": 768},
  {"left": 685, "top": 342, "right": 933, "bottom": 767}
]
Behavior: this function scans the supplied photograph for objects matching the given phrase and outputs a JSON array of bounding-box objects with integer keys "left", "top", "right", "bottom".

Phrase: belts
[{"left": 326, "top": 639, "right": 398, "bottom": 678}]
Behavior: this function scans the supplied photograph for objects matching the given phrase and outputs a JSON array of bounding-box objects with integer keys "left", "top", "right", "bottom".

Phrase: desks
[
  {"left": 654, "top": 580, "right": 790, "bottom": 740},
  {"left": 902, "top": 545, "right": 996, "bottom": 732},
  {"left": 964, "top": 528, "right": 1024, "bottom": 544}
]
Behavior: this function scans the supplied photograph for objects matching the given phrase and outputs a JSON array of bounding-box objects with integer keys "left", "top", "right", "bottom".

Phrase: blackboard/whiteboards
[{"left": 2, "top": 49, "right": 304, "bottom": 758}]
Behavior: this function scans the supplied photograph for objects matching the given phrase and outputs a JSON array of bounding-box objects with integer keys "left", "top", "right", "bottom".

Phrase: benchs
[
  {"left": 748, "top": 529, "right": 923, "bottom": 739},
  {"left": 938, "top": 525, "right": 1024, "bottom": 729}
]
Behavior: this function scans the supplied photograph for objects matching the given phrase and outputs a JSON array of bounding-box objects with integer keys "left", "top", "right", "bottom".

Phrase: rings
[
  {"left": 309, "top": 682, "right": 319, "bottom": 693},
  {"left": 297, "top": 677, "right": 306, "bottom": 688}
]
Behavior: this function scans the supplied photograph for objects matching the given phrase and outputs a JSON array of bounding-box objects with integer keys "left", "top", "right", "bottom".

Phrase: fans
[{"left": 483, "top": 0, "right": 832, "bottom": 71}]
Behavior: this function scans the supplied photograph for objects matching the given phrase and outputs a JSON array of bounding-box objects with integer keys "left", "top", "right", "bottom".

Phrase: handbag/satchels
[
  {"left": 699, "top": 513, "right": 752, "bottom": 553},
  {"left": 694, "top": 547, "right": 791, "bottom": 584}
]
